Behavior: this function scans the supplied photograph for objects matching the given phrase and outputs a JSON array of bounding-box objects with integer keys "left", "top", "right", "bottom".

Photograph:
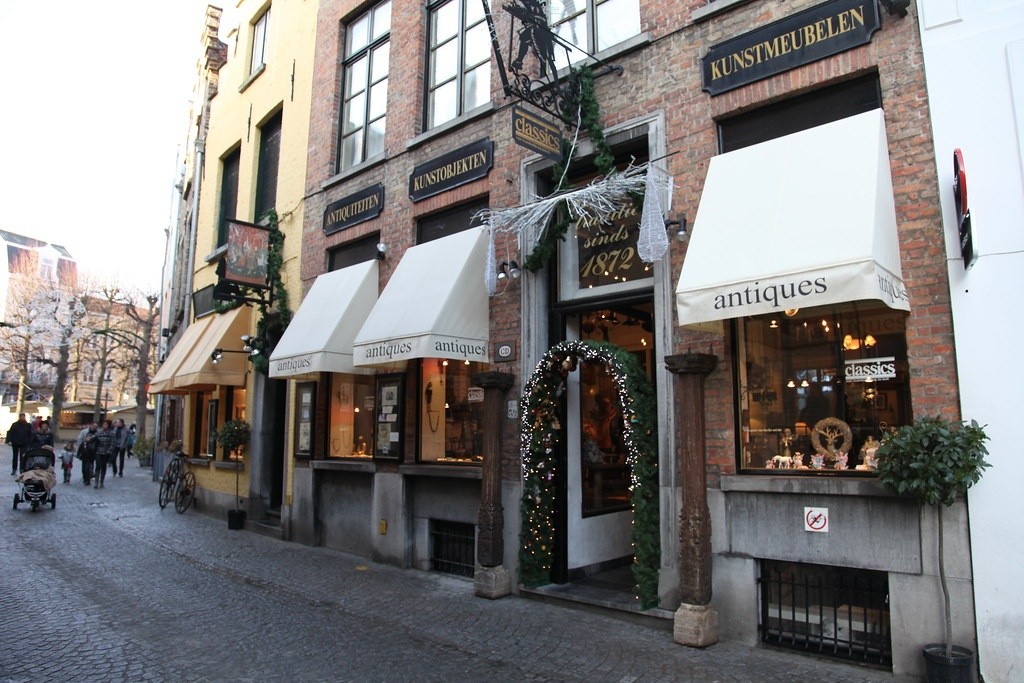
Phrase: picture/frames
[
  {"left": 294, "top": 380, "right": 317, "bottom": 459},
  {"left": 206, "top": 399, "right": 218, "bottom": 460},
  {"left": 373, "top": 372, "right": 405, "bottom": 461}
]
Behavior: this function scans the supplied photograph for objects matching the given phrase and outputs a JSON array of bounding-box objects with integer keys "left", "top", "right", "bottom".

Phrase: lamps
[
  {"left": 242, "top": 335, "right": 257, "bottom": 351},
  {"left": 212, "top": 348, "right": 252, "bottom": 364},
  {"left": 248, "top": 339, "right": 260, "bottom": 357}
]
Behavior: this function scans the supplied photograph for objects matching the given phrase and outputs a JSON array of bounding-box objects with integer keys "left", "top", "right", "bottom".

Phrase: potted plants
[
  {"left": 874, "top": 416, "right": 993, "bottom": 682},
  {"left": 133, "top": 434, "right": 155, "bottom": 467},
  {"left": 217, "top": 419, "right": 252, "bottom": 530}
]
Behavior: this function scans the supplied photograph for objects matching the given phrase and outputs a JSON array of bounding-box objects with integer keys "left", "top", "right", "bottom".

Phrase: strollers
[{"left": 12, "top": 444, "right": 57, "bottom": 513}]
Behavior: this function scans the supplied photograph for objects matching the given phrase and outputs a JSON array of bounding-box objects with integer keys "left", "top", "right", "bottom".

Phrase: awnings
[
  {"left": 676, "top": 108, "right": 914, "bottom": 339},
  {"left": 267, "top": 258, "right": 379, "bottom": 383},
  {"left": 351, "top": 223, "right": 495, "bottom": 370},
  {"left": 147, "top": 300, "right": 252, "bottom": 396}
]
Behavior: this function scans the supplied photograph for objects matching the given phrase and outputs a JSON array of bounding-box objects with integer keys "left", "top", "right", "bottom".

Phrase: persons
[
  {"left": 863, "top": 436, "right": 880, "bottom": 465},
  {"left": 30, "top": 415, "right": 59, "bottom": 434},
  {"left": 107, "top": 420, "right": 138, "bottom": 467},
  {"left": 58, "top": 441, "right": 82, "bottom": 485},
  {"left": 29, "top": 421, "right": 56, "bottom": 452},
  {"left": 78, "top": 434, "right": 98, "bottom": 487},
  {"left": 6, "top": 413, "right": 33, "bottom": 477},
  {"left": 92, "top": 419, "right": 116, "bottom": 489},
  {"left": 112, "top": 419, "right": 132, "bottom": 479},
  {"left": 78, "top": 422, "right": 101, "bottom": 479},
  {"left": 780, "top": 429, "right": 800, "bottom": 456}
]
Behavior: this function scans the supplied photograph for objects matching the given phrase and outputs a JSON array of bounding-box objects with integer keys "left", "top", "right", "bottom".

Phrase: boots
[
  {"left": 63, "top": 473, "right": 71, "bottom": 483},
  {"left": 94, "top": 471, "right": 106, "bottom": 488}
]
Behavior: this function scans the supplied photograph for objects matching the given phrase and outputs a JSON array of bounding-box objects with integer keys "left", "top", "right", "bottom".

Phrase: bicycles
[{"left": 158, "top": 449, "right": 197, "bottom": 514}]
